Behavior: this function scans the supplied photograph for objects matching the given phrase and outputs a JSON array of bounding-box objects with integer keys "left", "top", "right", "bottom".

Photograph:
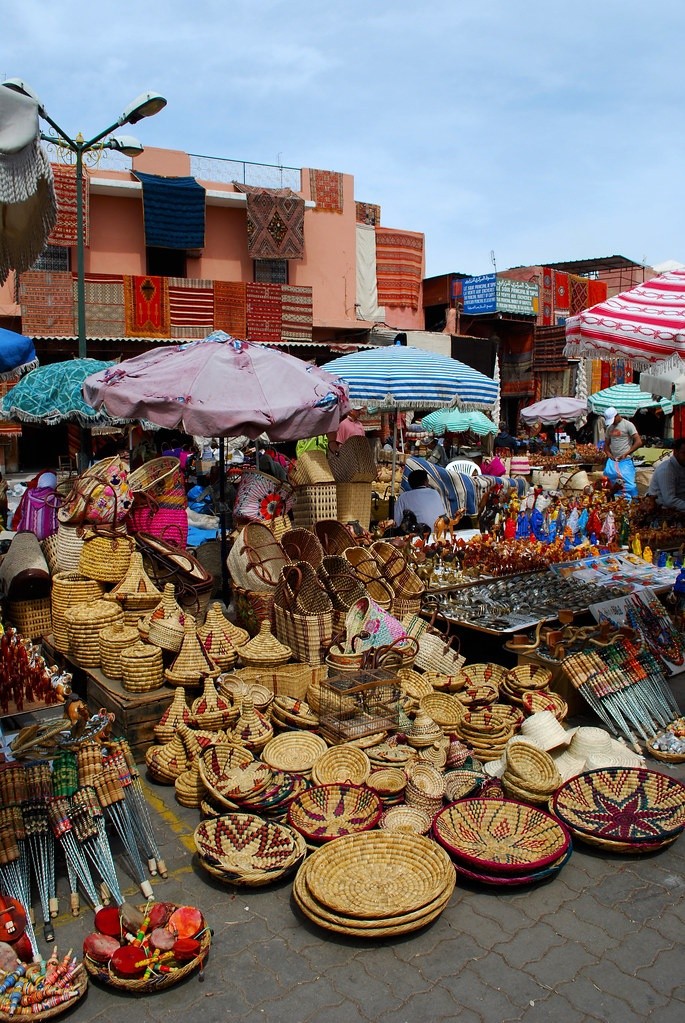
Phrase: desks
[{"left": 532, "top": 463, "right": 594, "bottom": 473}]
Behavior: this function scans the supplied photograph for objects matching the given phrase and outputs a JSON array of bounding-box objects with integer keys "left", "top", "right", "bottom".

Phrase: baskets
[
  {"left": 199, "top": 664, "right": 684, "bottom": 936},
  {"left": 1, "top": 454, "right": 212, "bottom": 628},
  {"left": 6, "top": 597, "right": 52, "bottom": 639},
  {"left": 82, "top": 903, "right": 210, "bottom": 990},
  {"left": 227, "top": 435, "right": 466, "bottom": 677},
  {"left": 559, "top": 471, "right": 593, "bottom": 495},
  {"left": 0, "top": 958, "right": 89, "bottom": 1021}
]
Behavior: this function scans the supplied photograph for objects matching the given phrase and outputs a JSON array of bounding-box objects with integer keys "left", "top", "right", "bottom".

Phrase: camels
[{"left": 433, "top": 508, "right": 467, "bottom": 539}]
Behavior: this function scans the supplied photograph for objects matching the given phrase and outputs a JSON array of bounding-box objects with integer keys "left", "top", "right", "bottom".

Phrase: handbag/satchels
[{"left": 482, "top": 457, "right": 506, "bottom": 477}]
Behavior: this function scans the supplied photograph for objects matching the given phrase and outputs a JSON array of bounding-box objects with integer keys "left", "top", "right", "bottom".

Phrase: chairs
[{"left": 445, "top": 459, "right": 482, "bottom": 476}]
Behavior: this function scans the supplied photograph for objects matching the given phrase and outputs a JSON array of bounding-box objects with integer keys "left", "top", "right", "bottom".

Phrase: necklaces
[{"left": 598, "top": 587, "right": 685, "bottom": 675}]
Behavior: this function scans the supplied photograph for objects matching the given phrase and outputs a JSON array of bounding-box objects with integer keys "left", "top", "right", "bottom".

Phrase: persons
[
  {"left": 603, "top": 406, "right": 643, "bottom": 496},
  {"left": 480, "top": 477, "right": 685, "bottom": 578},
  {"left": 337, "top": 404, "right": 364, "bottom": 449},
  {"left": 647, "top": 438, "right": 685, "bottom": 512},
  {"left": 591, "top": 411, "right": 607, "bottom": 449},
  {"left": 0, "top": 627, "right": 58, "bottom": 714},
  {"left": 384, "top": 434, "right": 399, "bottom": 453},
  {"left": 186, "top": 475, "right": 219, "bottom": 515},
  {"left": 535, "top": 437, "right": 560, "bottom": 456},
  {"left": 394, "top": 470, "right": 449, "bottom": 533},
  {"left": 10, "top": 471, "right": 63, "bottom": 540},
  {"left": 420, "top": 435, "right": 450, "bottom": 468},
  {"left": 162, "top": 438, "right": 202, "bottom": 480}
]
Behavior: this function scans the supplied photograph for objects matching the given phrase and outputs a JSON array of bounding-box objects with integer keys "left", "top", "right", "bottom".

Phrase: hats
[
  {"left": 603, "top": 407, "right": 617, "bottom": 425},
  {"left": 351, "top": 405, "right": 364, "bottom": 410}
]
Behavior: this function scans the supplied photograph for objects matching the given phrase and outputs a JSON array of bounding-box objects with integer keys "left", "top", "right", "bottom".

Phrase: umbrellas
[
  {"left": 0, "top": 358, "right": 119, "bottom": 466},
  {"left": 520, "top": 396, "right": 588, "bottom": 451},
  {"left": 588, "top": 382, "right": 673, "bottom": 423},
  {"left": 0, "top": 327, "right": 39, "bottom": 381},
  {"left": 316, "top": 342, "right": 501, "bottom": 521},
  {"left": 81, "top": 329, "right": 353, "bottom": 608},
  {"left": 563, "top": 266, "right": 685, "bottom": 375},
  {"left": 422, "top": 406, "right": 500, "bottom": 461}
]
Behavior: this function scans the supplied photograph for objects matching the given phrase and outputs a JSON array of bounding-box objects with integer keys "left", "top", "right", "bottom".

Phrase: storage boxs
[{"left": 517, "top": 654, "right": 588, "bottom": 716}]
